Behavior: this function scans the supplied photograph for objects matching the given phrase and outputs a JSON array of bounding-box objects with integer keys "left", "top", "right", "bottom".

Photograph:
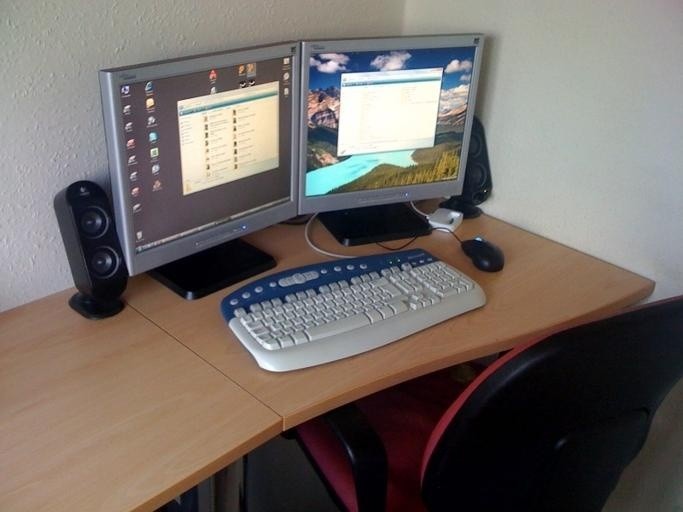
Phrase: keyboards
[{"left": 222, "top": 248, "right": 487, "bottom": 373}]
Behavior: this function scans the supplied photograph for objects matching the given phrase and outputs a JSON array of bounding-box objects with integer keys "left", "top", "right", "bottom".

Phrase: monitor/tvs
[
  {"left": 301, "top": 34, "right": 486, "bottom": 247},
  {"left": 98, "top": 38, "right": 300, "bottom": 301}
]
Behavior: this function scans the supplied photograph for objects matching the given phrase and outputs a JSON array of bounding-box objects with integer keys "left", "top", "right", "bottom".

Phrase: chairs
[{"left": 291, "top": 295, "right": 682, "bottom": 511}]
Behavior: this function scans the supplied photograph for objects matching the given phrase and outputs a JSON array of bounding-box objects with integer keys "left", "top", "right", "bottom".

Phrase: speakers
[
  {"left": 438, "top": 115, "right": 492, "bottom": 219},
  {"left": 53, "top": 180, "right": 129, "bottom": 320}
]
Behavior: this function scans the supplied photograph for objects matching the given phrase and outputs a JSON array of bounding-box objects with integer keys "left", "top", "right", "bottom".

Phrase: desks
[
  {"left": 1, "top": 285, "right": 283, "bottom": 511},
  {"left": 123, "top": 199, "right": 656, "bottom": 511}
]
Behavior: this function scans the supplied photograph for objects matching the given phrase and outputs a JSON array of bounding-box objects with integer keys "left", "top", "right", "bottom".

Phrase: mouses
[{"left": 463, "top": 240, "right": 504, "bottom": 272}]
